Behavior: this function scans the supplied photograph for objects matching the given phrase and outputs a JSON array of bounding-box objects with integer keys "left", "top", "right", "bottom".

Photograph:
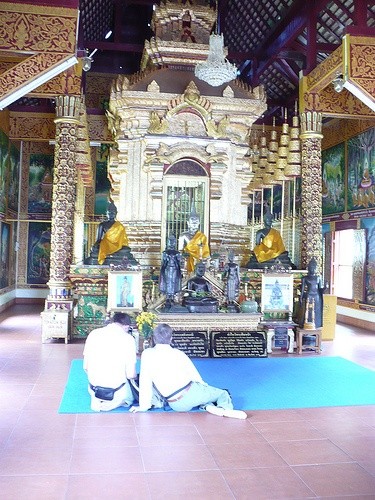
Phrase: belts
[{"left": 167, "top": 386, "right": 191, "bottom": 403}]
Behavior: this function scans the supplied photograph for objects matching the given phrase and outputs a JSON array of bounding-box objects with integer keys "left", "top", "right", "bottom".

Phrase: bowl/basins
[{"left": 240, "top": 300, "right": 258, "bottom": 313}]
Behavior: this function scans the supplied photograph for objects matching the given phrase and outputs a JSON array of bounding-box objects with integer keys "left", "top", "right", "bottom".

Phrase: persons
[
  {"left": 82, "top": 313, "right": 139, "bottom": 412},
  {"left": 89, "top": 202, "right": 131, "bottom": 263},
  {"left": 300, "top": 258, "right": 329, "bottom": 329},
  {"left": 253, "top": 207, "right": 286, "bottom": 263},
  {"left": 187, "top": 260, "right": 211, "bottom": 297},
  {"left": 178, "top": 212, "right": 210, "bottom": 274},
  {"left": 223, "top": 254, "right": 241, "bottom": 304},
  {"left": 158, "top": 234, "right": 183, "bottom": 301},
  {"left": 129, "top": 324, "right": 248, "bottom": 420}
]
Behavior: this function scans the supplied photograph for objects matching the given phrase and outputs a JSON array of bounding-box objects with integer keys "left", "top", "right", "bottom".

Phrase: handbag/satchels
[{"left": 93, "top": 387, "right": 114, "bottom": 401}]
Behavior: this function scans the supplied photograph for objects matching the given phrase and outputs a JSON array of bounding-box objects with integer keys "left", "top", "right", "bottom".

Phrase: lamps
[
  {"left": 332, "top": 74, "right": 346, "bottom": 92},
  {"left": 80, "top": 49, "right": 94, "bottom": 72},
  {"left": 194, "top": 0, "right": 239, "bottom": 88}
]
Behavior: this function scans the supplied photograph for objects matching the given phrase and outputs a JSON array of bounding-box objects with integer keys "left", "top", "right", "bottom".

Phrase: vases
[{"left": 142, "top": 340, "right": 150, "bottom": 349}]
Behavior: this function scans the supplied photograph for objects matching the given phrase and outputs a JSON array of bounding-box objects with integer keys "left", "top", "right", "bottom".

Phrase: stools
[
  {"left": 39, "top": 309, "right": 72, "bottom": 343},
  {"left": 296, "top": 326, "right": 323, "bottom": 355},
  {"left": 258, "top": 322, "right": 300, "bottom": 354}
]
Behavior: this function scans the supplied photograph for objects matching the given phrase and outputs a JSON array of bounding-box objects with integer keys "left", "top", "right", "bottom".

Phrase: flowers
[{"left": 135, "top": 310, "right": 160, "bottom": 339}]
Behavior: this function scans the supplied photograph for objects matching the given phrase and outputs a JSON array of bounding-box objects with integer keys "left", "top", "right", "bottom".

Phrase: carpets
[{"left": 54, "top": 357, "right": 375, "bottom": 415}]
